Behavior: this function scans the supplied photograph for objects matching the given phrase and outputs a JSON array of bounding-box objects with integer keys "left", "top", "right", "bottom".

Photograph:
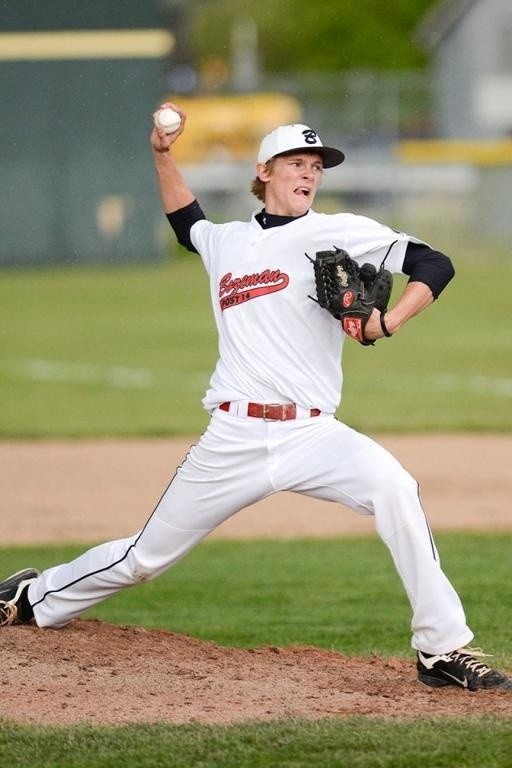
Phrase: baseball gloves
[{"left": 303, "top": 248, "right": 393, "bottom": 348}]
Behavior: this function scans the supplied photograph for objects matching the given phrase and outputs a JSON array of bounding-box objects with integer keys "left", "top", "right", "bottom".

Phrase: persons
[{"left": 1, "top": 96, "right": 512, "bottom": 695}]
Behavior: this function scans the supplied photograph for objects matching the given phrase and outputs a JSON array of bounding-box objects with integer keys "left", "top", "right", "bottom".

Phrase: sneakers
[
  {"left": 0, "top": 568, "right": 37, "bottom": 625},
  {"left": 416, "top": 646, "right": 512, "bottom": 691}
]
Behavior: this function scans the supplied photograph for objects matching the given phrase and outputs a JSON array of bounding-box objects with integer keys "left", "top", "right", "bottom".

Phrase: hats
[{"left": 259, "top": 124, "right": 344, "bottom": 169}]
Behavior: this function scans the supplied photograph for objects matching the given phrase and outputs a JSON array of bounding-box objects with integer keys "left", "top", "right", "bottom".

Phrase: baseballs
[{"left": 154, "top": 109, "right": 181, "bottom": 136}]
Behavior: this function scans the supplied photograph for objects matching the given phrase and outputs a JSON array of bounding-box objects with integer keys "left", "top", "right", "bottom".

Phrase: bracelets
[{"left": 377, "top": 306, "right": 390, "bottom": 340}]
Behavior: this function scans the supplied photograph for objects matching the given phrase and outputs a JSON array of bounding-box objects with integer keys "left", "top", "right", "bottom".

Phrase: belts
[{"left": 219, "top": 402, "right": 320, "bottom": 419}]
